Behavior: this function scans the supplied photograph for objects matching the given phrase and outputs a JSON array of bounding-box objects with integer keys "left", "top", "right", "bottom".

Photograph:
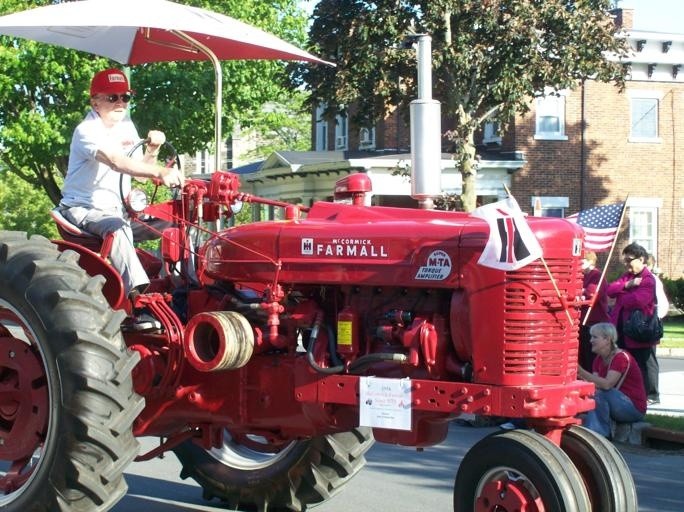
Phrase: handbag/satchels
[{"left": 621, "top": 309, "right": 665, "bottom": 343}]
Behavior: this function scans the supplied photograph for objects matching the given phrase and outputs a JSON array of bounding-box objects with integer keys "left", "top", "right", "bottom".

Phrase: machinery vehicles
[{"left": 0, "top": 0, "right": 638, "bottom": 512}]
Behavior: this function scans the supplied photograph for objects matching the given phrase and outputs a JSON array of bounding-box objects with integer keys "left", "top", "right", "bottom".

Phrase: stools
[{"left": 51, "top": 208, "right": 125, "bottom": 253}]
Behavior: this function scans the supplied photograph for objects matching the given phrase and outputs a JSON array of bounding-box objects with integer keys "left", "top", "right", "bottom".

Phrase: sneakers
[{"left": 132, "top": 306, "right": 162, "bottom": 331}]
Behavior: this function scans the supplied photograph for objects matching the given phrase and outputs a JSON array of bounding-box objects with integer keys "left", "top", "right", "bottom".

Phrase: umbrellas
[{"left": 0, "top": 0, "right": 338, "bottom": 234}]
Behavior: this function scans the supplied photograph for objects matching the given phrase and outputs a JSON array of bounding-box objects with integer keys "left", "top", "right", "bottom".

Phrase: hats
[{"left": 90, "top": 69, "right": 134, "bottom": 97}]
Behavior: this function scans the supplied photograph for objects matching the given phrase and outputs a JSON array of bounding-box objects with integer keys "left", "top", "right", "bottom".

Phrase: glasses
[{"left": 95, "top": 94, "right": 131, "bottom": 102}]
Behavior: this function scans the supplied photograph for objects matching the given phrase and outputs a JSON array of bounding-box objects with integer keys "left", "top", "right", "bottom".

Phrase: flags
[
  {"left": 559, "top": 198, "right": 627, "bottom": 251},
  {"left": 470, "top": 196, "right": 544, "bottom": 271}
]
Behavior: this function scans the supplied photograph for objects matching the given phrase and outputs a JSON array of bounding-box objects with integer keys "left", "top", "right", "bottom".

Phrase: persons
[
  {"left": 57, "top": 69, "right": 181, "bottom": 332},
  {"left": 576, "top": 241, "right": 669, "bottom": 442}
]
